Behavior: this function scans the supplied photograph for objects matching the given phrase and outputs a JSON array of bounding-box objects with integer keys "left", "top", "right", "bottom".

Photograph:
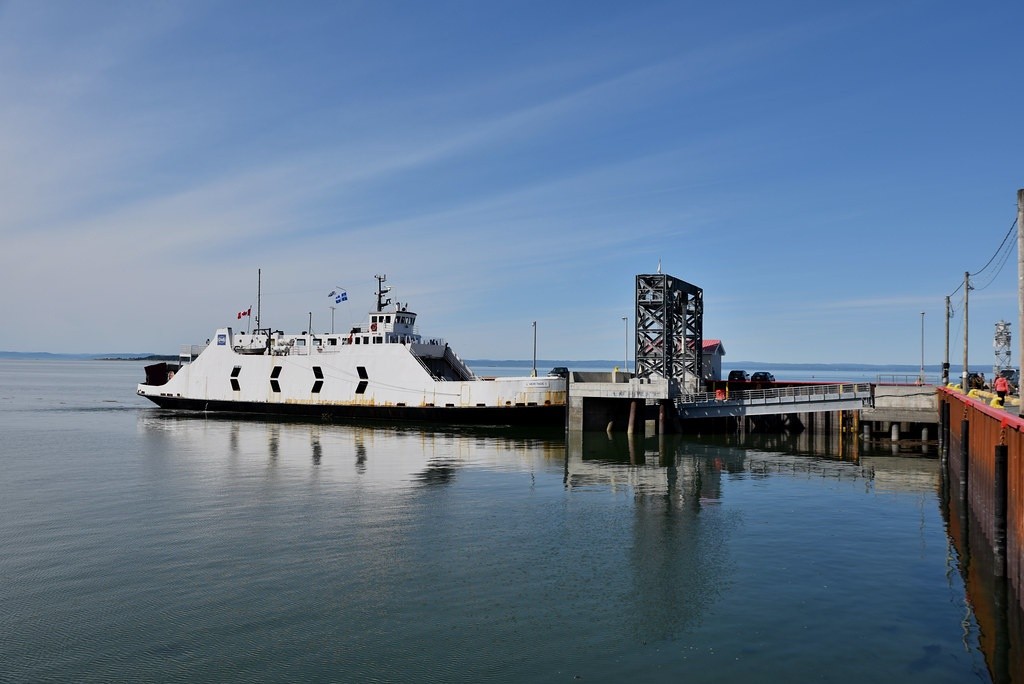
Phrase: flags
[
  {"left": 335, "top": 292, "right": 347, "bottom": 302},
  {"left": 657, "top": 261, "right": 661, "bottom": 272},
  {"left": 237, "top": 309, "right": 251, "bottom": 319}
]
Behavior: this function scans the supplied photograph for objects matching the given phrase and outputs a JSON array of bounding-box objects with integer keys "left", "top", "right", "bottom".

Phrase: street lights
[
  {"left": 531, "top": 322, "right": 538, "bottom": 375},
  {"left": 921, "top": 312, "right": 925, "bottom": 384},
  {"left": 623, "top": 316, "right": 628, "bottom": 373}
]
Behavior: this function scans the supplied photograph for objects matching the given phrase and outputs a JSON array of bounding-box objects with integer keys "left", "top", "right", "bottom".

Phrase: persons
[
  {"left": 614, "top": 366, "right": 619, "bottom": 371},
  {"left": 995, "top": 373, "right": 1008, "bottom": 406},
  {"left": 916, "top": 378, "right": 922, "bottom": 385},
  {"left": 993, "top": 374, "right": 1000, "bottom": 386},
  {"left": 168, "top": 370, "right": 174, "bottom": 379},
  {"left": 429, "top": 339, "right": 438, "bottom": 345}
]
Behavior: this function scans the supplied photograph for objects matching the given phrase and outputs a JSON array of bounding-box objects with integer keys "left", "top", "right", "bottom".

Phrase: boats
[{"left": 135, "top": 268, "right": 568, "bottom": 438}]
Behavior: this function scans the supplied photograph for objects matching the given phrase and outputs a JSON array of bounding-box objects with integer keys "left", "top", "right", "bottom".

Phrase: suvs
[{"left": 547, "top": 367, "right": 568, "bottom": 379}]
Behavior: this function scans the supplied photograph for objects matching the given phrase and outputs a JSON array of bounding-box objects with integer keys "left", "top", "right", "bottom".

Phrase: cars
[
  {"left": 959, "top": 373, "right": 984, "bottom": 390},
  {"left": 752, "top": 372, "right": 775, "bottom": 382},
  {"left": 728, "top": 370, "right": 751, "bottom": 382}
]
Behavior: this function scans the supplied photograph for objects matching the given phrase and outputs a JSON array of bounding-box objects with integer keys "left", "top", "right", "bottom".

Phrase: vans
[{"left": 999, "top": 369, "right": 1019, "bottom": 388}]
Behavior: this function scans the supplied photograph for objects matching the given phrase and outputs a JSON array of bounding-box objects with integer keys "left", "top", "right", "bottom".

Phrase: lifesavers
[{"left": 371, "top": 324, "right": 377, "bottom": 331}]
